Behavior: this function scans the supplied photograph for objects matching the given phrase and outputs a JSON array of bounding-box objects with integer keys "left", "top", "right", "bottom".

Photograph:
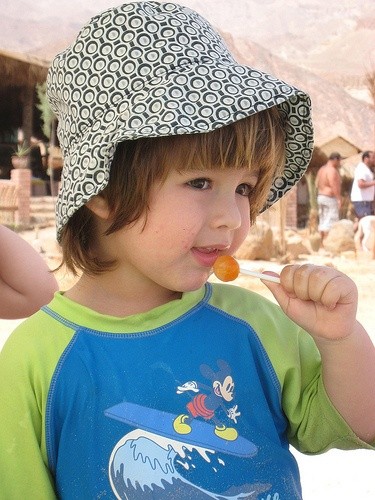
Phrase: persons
[
  {"left": 315, "top": 151, "right": 342, "bottom": 241},
  {"left": 0, "top": 2, "right": 375, "bottom": 500},
  {"left": 0, "top": 224, "right": 58, "bottom": 320},
  {"left": 351, "top": 151, "right": 374, "bottom": 220}
]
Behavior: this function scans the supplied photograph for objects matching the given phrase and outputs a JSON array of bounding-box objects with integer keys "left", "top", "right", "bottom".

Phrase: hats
[{"left": 46, "top": 1, "right": 314, "bottom": 239}]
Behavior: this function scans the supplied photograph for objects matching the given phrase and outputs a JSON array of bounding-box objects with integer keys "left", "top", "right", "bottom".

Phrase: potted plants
[{"left": 10, "top": 140, "right": 32, "bottom": 169}]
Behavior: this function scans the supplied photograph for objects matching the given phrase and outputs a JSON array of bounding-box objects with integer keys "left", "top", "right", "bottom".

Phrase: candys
[{"left": 212, "top": 255, "right": 280, "bottom": 285}]
